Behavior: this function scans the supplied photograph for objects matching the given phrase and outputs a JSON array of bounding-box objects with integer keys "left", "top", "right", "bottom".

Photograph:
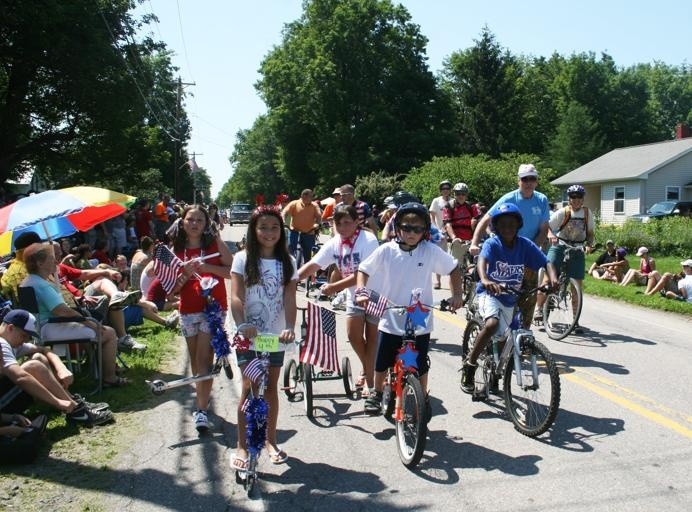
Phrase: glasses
[
  {"left": 441, "top": 187, "right": 450, "bottom": 190},
  {"left": 570, "top": 195, "right": 583, "bottom": 199},
  {"left": 521, "top": 176, "right": 536, "bottom": 182},
  {"left": 400, "top": 224, "right": 424, "bottom": 233}
]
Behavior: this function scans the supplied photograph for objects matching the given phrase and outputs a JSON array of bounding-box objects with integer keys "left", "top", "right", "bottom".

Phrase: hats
[
  {"left": 519, "top": 164, "right": 537, "bottom": 177},
  {"left": 14, "top": 232, "right": 47, "bottom": 249},
  {"left": 3, "top": 309, "right": 40, "bottom": 339},
  {"left": 616, "top": 248, "right": 626, "bottom": 257},
  {"left": 636, "top": 246, "right": 648, "bottom": 256},
  {"left": 681, "top": 259, "right": 692, "bottom": 266}
]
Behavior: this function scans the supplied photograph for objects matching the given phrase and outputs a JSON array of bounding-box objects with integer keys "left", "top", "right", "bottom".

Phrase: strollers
[{"left": 303, "top": 241, "right": 332, "bottom": 301}]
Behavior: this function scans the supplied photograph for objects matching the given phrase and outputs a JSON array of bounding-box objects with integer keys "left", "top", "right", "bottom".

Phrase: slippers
[
  {"left": 233, "top": 456, "right": 249, "bottom": 471},
  {"left": 269, "top": 449, "right": 289, "bottom": 464}
]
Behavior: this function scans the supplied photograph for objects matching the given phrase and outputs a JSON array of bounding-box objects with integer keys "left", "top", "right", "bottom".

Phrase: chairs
[{"left": 16, "top": 284, "right": 104, "bottom": 394}]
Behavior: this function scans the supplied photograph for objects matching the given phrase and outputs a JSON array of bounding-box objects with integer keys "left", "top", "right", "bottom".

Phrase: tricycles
[{"left": 278, "top": 303, "right": 357, "bottom": 419}]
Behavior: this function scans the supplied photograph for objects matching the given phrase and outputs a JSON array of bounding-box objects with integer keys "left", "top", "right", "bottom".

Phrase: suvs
[
  {"left": 229, "top": 203, "right": 253, "bottom": 227},
  {"left": 626, "top": 197, "right": 692, "bottom": 223}
]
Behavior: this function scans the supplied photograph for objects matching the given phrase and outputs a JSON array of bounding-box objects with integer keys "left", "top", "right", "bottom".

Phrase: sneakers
[
  {"left": 118, "top": 334, "right": 147, "bottom": 351},
  {"left": 67, "top": 393, "right": 113, "bottom": 426},
  {"left": 461, "top": 358, "right": 479, "bottom": 393},
  {"left": 105, "top": 377, "right": 132, "bottom": 388},
  {"left": 364, "top": 391, "right": 383, "bottom": 413},
  {"left": 192, "top": 400, "right": 210, "bottom": 430}
]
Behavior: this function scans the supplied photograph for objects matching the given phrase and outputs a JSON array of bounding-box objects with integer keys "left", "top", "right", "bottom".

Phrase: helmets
[
  {"left": 567, "top": 184, "right": 585, "bottom": 193},
  {"left": 440, "top": 180, "right": 451, "bottom": 191},
  {"left": 490, "top": 202, "right": 524, "bottom": 229},
  {"left": 385, "top": 191, "right": 431, "bottom": 232},
  {"left": 453, "top": 183, "right": 468, "bottom": 192}
]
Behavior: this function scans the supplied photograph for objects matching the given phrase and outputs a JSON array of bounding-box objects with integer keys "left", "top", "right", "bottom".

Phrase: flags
[{"left": 186, "top": 159, "right": 198, "bottom": 170}]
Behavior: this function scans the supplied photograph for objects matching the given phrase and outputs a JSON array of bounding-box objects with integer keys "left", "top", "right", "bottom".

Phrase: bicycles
[
  {"left": 361, "top": 291, "right": 456, "bottom": 472},
  {"left": 449, "top": 239, "right": 484, "bottom": 306},
  {"left": 540, "top": 235, "right": 589, "bottom": 342},
  {"left": 456, "top": 278, "right": 567, "bottom": 440},
  {"left": 283, "top": 219, "right": 330, "bottom": 270}
]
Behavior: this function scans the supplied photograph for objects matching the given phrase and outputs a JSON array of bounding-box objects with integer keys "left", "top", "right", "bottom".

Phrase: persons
[
  {"left": 588, "top": 239, "right": 692, "bottom": 306},
  {"left": 1, "top": 163, "right": 596, "bottom": 474}
]
